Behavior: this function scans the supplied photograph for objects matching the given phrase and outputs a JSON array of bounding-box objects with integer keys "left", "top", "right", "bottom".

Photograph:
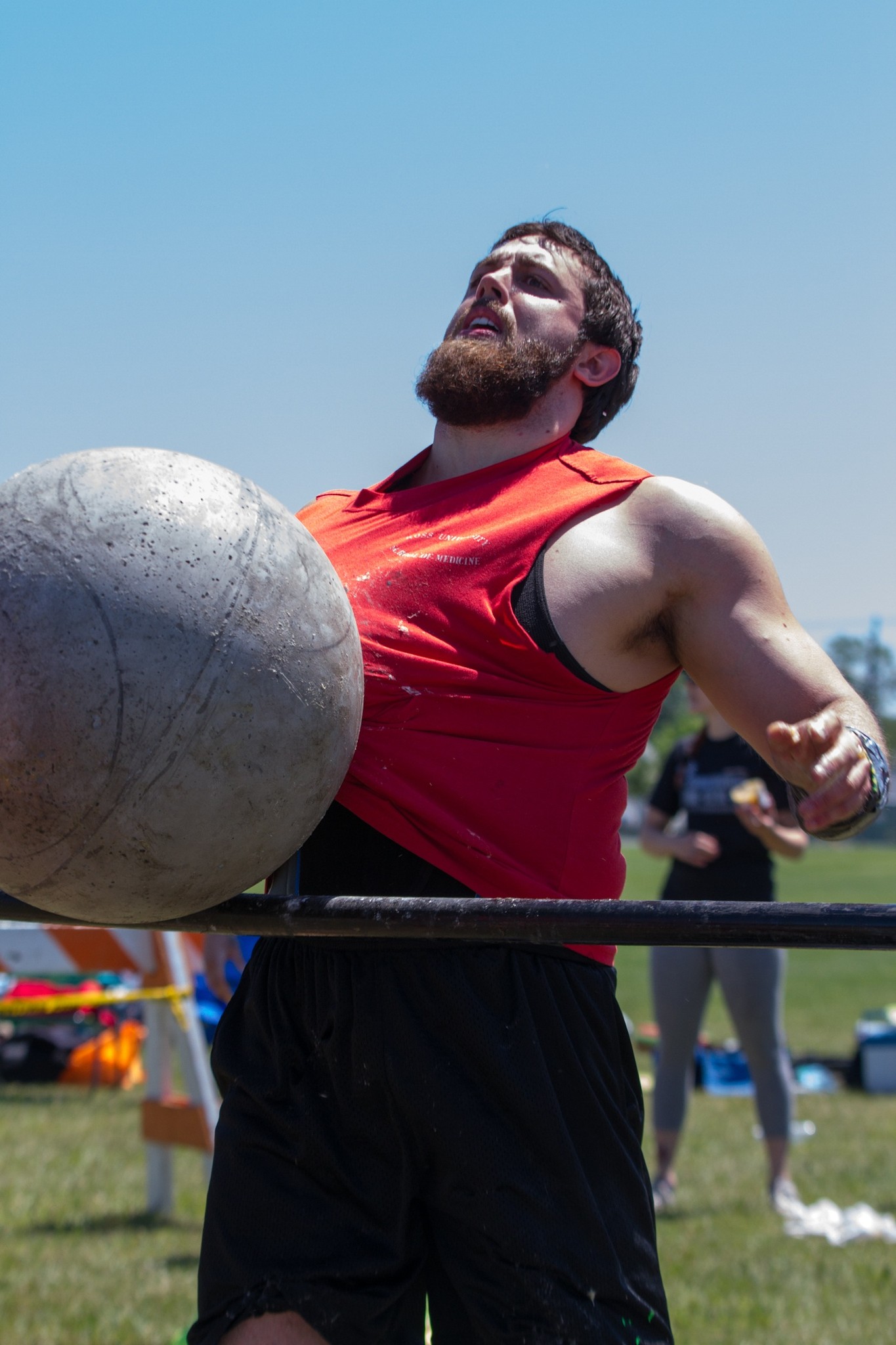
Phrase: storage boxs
[{"left": 854, "top": 1016, "right": 896, "bottom": 1093}]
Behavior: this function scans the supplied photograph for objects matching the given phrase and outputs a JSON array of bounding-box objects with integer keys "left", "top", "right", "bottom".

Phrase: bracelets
[{"left": 791, "top": 727, "right": 887, "bottom": 838}]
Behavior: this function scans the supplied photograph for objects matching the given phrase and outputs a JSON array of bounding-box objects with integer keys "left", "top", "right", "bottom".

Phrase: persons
[
  {"left": 185, "top": 219, "right": 896, "bottom": 1345},
  {"left": 624, "top": 666, "right": 806, "bottom": 1216}
]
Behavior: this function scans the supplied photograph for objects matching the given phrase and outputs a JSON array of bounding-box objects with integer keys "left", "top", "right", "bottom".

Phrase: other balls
[{"left": 2, "top": 445, "right": 367, "bottom": 930}]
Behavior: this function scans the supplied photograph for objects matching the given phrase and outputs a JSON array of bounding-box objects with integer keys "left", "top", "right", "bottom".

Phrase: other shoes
[
  {"left": 650, "top": 1175, "right": 677, "bottom": 1214},
  {"left": 769, "top": 1177, "right": 804, "bottom": 1220}
]
